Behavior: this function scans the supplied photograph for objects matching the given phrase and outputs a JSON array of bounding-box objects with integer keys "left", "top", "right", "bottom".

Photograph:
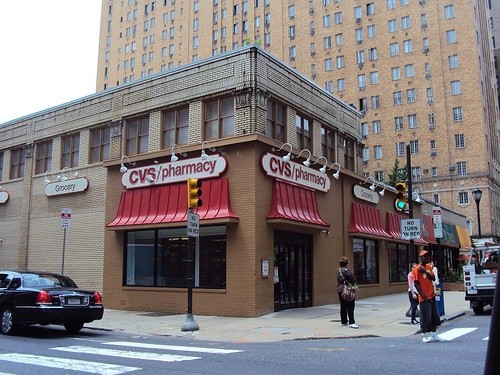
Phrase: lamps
[
  {"left": 374, "top": 182, "right": 386, "bottom": 196},
  {"left": 294, "top": 149, "right": 311, "bottom": 167},
  {"left": 359, "top": 176, "right": 376, "bottom": 190},
  {"left": 201, "top": 142, "right": 217, "bottom": 159},
  {"left": 44, "top": 170, "right": 60, "bottom": 185},
  {"left": 312, "top": 156, "right": 328, "bottom": 173},
  {"left": 170, "top": 144, "right": 187, "bottom": 161},
  {"left": 61, "top": 166, "right": 79, "bottom": 181},
  {"left": 327, "top": 164, "right": 341, "bottom": 179},
  {"left": 274, "top": 143, "right": 292, "bottom": 161},
  {"left": 411, "top": 191, "right": 421, "bottom": 203},
  {"left": 120, "top": 156, "right": 136, "bottom": 173},
  {"left": 145, "top": 160, "right": 162, "bottom": 183}
]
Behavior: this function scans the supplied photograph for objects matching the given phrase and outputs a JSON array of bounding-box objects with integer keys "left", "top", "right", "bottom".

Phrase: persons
[
  {"left": 407, "top": 263, "right": 420, "bottom": 325},
  {"left": 413, "top": 250, "right": 441, "bottom": 343},
  {"left": 430, "top": 259, "right": 439, "bottom": 294},
  {"left": 337, "top": 256, "right": 359, "bottom": 328}
]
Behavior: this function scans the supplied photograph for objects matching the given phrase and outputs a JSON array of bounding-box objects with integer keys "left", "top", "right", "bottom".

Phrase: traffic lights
[
  {"left": 187, "top": 178, "right": 203, "bottom": 209},
  {"left": 395, "top": 182, "right": 408, "bottom": 210}
]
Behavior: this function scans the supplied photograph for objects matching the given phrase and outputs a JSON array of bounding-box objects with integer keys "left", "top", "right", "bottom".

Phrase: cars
[{"left": 0, "top": 269, "right": 105, "bottom": 335}]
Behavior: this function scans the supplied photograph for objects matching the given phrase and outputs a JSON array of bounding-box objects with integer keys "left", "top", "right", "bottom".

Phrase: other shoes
[
  {"left": 410, "top": 319, "right": 420, "bottom": 324},
  {"left": 430, "top": 336, "right": 444, "bottom": 342},
  {"left": 348, "top": 323, "right": 359, "bottom": 328},
  {"left": 422, "top": 337, "right": 430, "bottom": 343},
  {"left": 341, "top": 322, "right": 349, "bottom": 326}
]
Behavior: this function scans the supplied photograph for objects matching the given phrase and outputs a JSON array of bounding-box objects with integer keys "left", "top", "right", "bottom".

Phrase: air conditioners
[
  {"left": 431, "top": 152, "right": 436, "bottom": 157},
  {"left": 420, "top": 23, "right": 426, "bottom": 28},
  {"left": 425, "top": 73, "right": 430, "bottom": 78},
  {"left": 426, "top": 99, "right": 432, "bottom": 103},
  {"left": 449, "top": 166, "right": 455, "bottom": 171},
  {"left": 423, "top": 168, "right": 429, "bottom": 174},
  {"left": 429, "top": 125, "right": 434, "bottom": 129},
  {"left": 419, "top": 0, "right": 424, "bottom": 4},
  {"left": 424, "top": 48, "right": 429, "bottom": 53},
  {"left": 104, "top": 0, "right": 367, "bottom": 114}
]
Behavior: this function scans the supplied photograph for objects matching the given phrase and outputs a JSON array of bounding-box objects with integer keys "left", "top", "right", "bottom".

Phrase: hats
[{"left": 419, "top": 250, "right": 429, "bottom": 257}]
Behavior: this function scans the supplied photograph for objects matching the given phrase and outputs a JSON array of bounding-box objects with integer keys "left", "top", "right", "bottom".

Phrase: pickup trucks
[{"left": 461, "top": 241, "right": 500, "bottom": 316}]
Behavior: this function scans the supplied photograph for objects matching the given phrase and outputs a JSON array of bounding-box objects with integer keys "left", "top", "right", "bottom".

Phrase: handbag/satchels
[
  {"left": 341, "top": 285, "right": 356, "bottom": 302},
  {"left": 351, "top": 283, "right": 360, "bottom": 295}
]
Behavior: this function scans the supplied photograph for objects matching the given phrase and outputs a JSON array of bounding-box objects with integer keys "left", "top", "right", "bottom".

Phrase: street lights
[{"left": 470, "top": 187, "right": 483, "bottom": 238}]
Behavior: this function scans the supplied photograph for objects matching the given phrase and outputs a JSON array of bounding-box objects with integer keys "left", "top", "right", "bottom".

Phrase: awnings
[
  {"left": 440, "top": 222, "right": 459, "bottom": 248},
  {"left": 454, "top": 225, "right": 472, "bottom": 248}
]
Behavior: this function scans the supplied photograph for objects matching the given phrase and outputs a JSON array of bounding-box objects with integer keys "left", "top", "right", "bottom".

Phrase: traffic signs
[{"left": 187, "top": 212, "right": 200, "bottom": 237}]
